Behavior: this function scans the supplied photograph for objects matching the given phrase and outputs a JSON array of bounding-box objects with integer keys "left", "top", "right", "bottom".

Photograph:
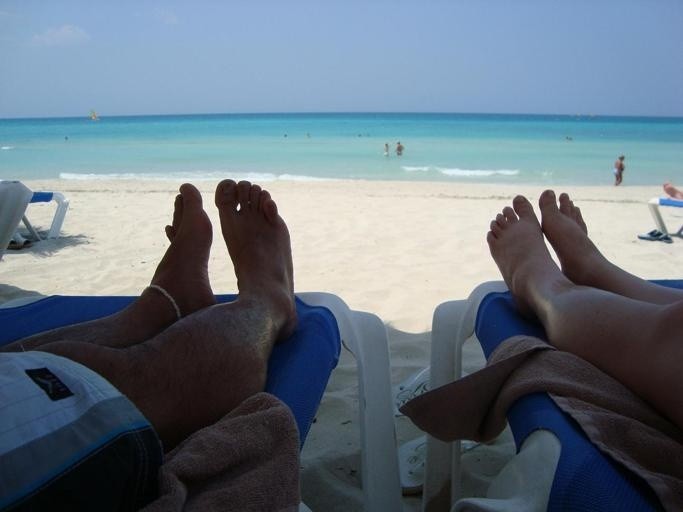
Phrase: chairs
[
  {"left": 21, "top": 192, "right": 69, "bottom": 241},
  {"left": 647, "top": 197, "right": 683, "bottom": 235},
  {"left": 0, "top": 292, "right": 403, "bottom": 512},
  {"left": 422, "top": 280, "right": 683, "bottom": 512}
]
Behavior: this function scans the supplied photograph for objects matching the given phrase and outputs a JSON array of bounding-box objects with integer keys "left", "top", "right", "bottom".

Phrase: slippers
[
  {"left": 638, "top": 229, "right": 671, "bottom": 241},
  {"left": 7, "top": 233, "right": 33, "bottom": 249}
]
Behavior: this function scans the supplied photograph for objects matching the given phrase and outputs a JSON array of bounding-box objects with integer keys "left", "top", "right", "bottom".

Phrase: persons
[
  {"left": 485, "top": 188, "right": 683, "bottom": 428},
  {"left": 383, "top": 143, "right": 389, "bottom": 156},
  {"left": 0, "top": 178, "right": 300, "bottom": 512},
  {"left": 614, "top": 155, "right": 625, "bottom": 185},
  {"left": 395, "top": 141, "right": 404, "bottom": 156},
  {"left": 664, "top": 182, "right": 683, "bottom": 200}
]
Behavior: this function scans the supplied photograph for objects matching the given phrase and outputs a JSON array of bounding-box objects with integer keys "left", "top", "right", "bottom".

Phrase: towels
[
  {"left": 399, "top": 335, "right": 683, "bottom": 512},
  {"left": 138, "top": 391, "right": 301, "bottom": 512}
]
